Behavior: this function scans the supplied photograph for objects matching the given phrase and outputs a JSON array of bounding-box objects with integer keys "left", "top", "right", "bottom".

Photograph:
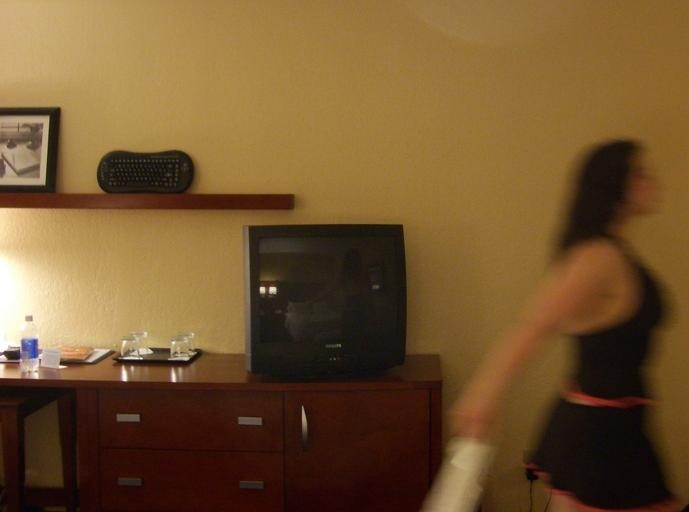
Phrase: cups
[
  {"left": 119, "top": 332, "right": 152, "bottom": 359},
  {"left": 42, "top": 349, "right": 60, "bottom": 368},
  {"left": 169, "top": 330, "right": 192, "bottom": 360}
]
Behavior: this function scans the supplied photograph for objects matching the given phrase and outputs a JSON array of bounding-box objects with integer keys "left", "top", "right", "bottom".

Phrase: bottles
[{"left": 19, "top": 314, "right": 38, "bottom": 370}]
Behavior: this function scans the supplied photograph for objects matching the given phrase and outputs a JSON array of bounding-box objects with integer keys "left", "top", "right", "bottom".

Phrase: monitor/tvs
[{"left": 248, "top": 224, "right": 407, "bottom": 378}]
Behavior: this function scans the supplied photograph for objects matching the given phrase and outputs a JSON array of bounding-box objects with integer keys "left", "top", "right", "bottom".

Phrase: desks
[{"left": 0, "top": 351, "right": 446, "bottom": 512}]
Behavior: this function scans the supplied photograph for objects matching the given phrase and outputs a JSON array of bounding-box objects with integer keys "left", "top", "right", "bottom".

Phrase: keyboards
[{"left": 97, "top": 150, "right": 194, "bottom": 193}]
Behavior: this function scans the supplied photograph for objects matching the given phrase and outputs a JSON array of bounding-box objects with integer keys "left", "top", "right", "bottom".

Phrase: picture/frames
[{"left": 0, "top": 106, "right": 63, "bottom": 193}]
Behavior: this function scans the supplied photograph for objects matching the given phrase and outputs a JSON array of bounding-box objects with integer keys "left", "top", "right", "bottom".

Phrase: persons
[{"left": 438, "top": 137, "right": 686, "bottom": 510}]
